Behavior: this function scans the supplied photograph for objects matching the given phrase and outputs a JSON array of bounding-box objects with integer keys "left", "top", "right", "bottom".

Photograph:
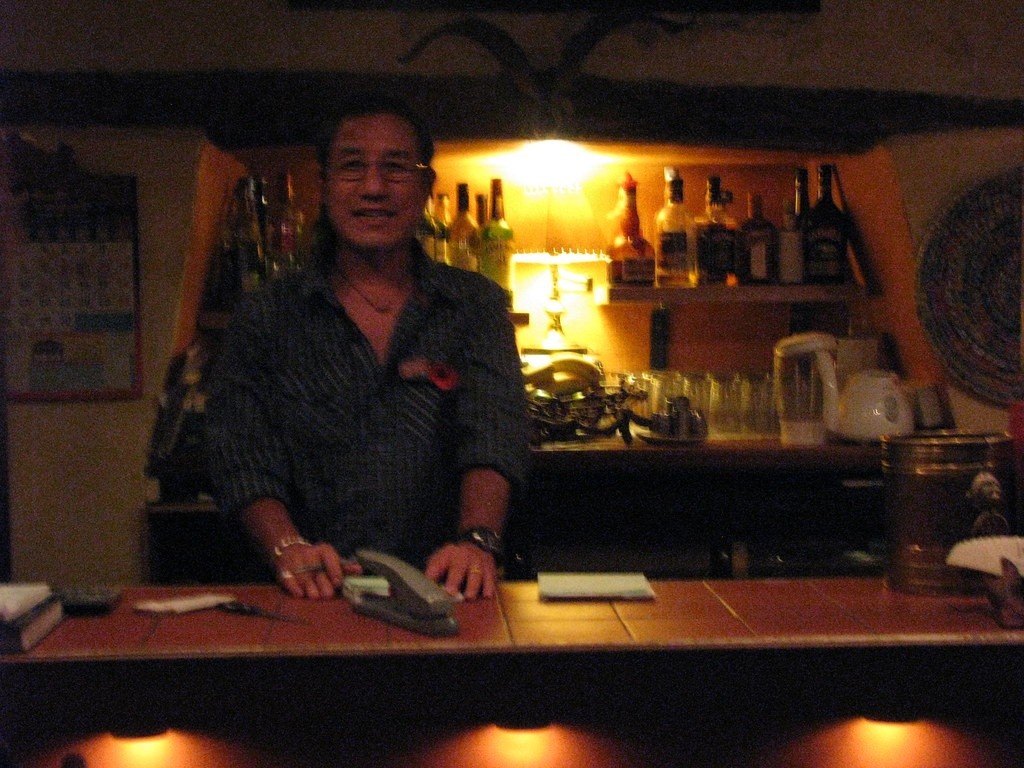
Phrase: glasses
[{"left": 323, "top": 158, "right": 428, "bottom": 184}]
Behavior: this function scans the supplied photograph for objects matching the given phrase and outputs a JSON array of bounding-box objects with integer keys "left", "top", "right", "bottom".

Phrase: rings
[{"left": 467, "top": 567, "right": 484, "bottom": 576}]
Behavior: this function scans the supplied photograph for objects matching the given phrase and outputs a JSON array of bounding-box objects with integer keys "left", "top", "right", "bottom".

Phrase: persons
[{"left": 203, "top": 94, "right": 534, "bottom": 601}]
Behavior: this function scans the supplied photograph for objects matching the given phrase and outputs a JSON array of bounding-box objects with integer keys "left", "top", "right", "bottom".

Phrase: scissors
[{"left": 218, "top": 601, "right": 301, "bottom": 621}]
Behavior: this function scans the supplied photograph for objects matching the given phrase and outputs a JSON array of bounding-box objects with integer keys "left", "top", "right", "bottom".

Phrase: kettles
[{"left": 772, "top": 331, "right": 843, "bottom": 449}]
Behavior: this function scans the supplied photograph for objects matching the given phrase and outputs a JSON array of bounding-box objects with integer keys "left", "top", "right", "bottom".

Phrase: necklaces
[{"left": 336, "top": 259, "right": 407, "bottom": 311}]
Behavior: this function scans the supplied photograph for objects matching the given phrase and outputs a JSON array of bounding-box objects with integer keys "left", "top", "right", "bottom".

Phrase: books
[{"left": 0, "top": 583, "right": 68, "bottom": 654}]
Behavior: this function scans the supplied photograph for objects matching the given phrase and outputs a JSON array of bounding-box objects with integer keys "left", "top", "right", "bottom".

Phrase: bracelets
[{"left": 269, "top": 535, "right": 313, "bottom": 573}]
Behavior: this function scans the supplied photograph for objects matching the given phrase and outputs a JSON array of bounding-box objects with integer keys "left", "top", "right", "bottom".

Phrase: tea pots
[{"left": 834, "top": 370, "right": 913, "bottom": 445}]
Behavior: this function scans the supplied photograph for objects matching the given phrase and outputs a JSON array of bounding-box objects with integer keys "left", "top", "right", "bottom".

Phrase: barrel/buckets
[{"left": 879, "top": 432, "right": 1014, "bottom": 594}]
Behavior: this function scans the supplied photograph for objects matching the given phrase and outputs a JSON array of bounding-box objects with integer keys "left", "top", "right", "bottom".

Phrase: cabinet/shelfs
[{"left": 148, "top": 285, "right": 888, "bottom": 578}]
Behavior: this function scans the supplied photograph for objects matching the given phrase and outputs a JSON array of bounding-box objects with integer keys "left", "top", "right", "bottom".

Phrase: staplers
[{"left": 352, "top": 549, "right": 460, "bottom": 636}]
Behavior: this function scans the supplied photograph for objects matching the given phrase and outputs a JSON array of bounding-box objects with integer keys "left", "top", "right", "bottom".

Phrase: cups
[{"left": 622, "top": 367, "right": 773, "bottom": 437}]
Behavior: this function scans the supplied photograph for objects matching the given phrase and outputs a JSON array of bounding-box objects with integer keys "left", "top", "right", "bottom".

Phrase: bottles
[
  {"left": 655, "top": 179, "right": 695, "bottom": 289},
  {"left": 420, "top": 179, "right": 512, "bottom": 294},
  {"left": 720, "top": 163, "right": 848, "bottom": 286},
  {"left": 695, "top": 175, "right": 735, "bottom": 288},
  {"left": 608, "top": 182, "right": 655, "bottom": 285},
  {"left": 217, "top": 167, "right": 304, "bottom": 304}
]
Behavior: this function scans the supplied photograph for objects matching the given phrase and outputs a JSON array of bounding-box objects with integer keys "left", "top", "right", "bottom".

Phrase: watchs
[{"left": 455, "top": 525, "right": 506, "bottom": 565}]
